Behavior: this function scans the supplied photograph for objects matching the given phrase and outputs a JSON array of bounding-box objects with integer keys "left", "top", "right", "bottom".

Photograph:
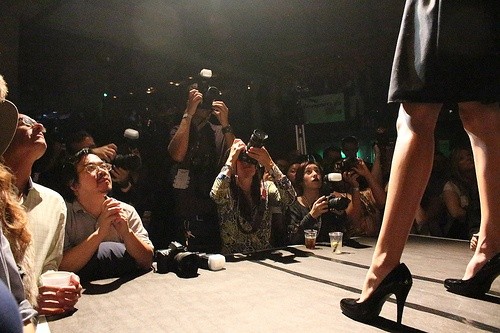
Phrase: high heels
[
  {"left": 444, "top": 252, "right": 500, "bottom": 297},
  {"left": 339, "top": 262, "right": 412, "bottom": 327}
]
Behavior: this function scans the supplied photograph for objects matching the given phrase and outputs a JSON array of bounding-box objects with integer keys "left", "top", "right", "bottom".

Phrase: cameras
[
  {"left": 326, "top": 196, "right": 350, "bottom": 212},
  {"left": 322, "top": 173, "right": 342, "bottom": 193},
  {"left": 336, "top": 154, "right": 359, "bottom": 171},
  {"left": 106, "top": 154, "right": 141, "bottom": 170},
  {"left": 197, "top": 69, "right": 222, "bottom": 108},
  {"left": 240, "top": 128, "right": 268, "bottom": 164},
  {"left": 156, "top": 240, "right": 199, "bottom": 279}
]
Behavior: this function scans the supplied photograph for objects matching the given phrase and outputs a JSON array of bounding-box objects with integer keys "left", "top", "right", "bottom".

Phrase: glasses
[
  {"left": 76, "top": 163, "right": 113, "bottom": 175},
  {"left": 19, "top": 116, "right": 47, "bottom": 134}
]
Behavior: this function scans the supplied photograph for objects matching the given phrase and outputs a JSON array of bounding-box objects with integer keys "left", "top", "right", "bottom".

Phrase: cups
[
  {"left": 304, "top": 229, "right": 317, "bottom": 250},
  {"left": 38, "top": 271, "right": 72, "bottom": 312},
  {"left": 329, "top": 232, "right": 343, "bottom": 254}
]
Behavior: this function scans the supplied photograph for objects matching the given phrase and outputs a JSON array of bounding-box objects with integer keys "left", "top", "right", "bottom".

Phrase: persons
[
  {"left": 0, "top": 74, "right": 500, "bottom": 333},
  {"left": 338, "top": 0, "right": 500, "bottom": 327}
]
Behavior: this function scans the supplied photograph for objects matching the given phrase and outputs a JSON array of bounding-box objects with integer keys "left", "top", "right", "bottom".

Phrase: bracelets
[
  {"left": 222, "top": 124, "right": 234, "bottom": 134},
  {"left": 183, "top": 113, "right": 192, "bottom": 119}
]
